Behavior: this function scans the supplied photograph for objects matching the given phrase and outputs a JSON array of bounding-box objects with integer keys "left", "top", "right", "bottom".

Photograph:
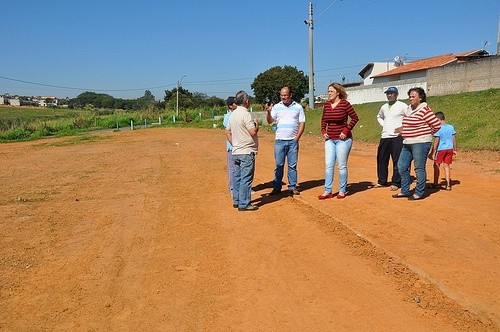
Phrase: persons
[
  {"left": 223, "top": 96, "right": 237, "bottom": 197},
  {"left": 392, "top": 87, "right": 442, "bottom": 201},
  {"left": 226, "top": 90, "right": 259, "bottom": 211},
  {"left": 318, "top": 82, "right": 359, "bottom": 199},
  {"left": 428, "top": 111, "right": 457, "bottom": 191},
  {"left": 373, "top": 87, "right": 409, "bottom": 191},
  {"left": 265, "top": 86, "right": 306, "bottom": 196}
]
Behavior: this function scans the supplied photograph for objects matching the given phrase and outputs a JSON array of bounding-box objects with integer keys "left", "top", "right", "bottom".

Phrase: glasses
[{"left": 386, "top": 93, "right": 392, "bottom": 95}]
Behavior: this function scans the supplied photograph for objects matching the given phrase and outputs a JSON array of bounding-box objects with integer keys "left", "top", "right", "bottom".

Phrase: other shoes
[
  {"left": 289, "top": 190, "right": 300, "bottom": 195},
  {"left": 441, "top": 185, "right": 451, "bottom": 190},
  {"left": 337, "top": 195, "right": 345, "bottom": 199},
  {"left": 428, "top": 184, "right": 438, "bottom": 188},
  {"left": 408, "top": 193, "right": 423, "bottom": 199},
  {"left": 233, "top": 204, "right": 238, "bottom": 208},
  {"left": 390, "top": 185, "right": 398, "bottom": 190},
  {"left": 238, "top": 205, "right": 258, "bottom": 211},
  {"left": 393, "top": 193, "right": 410, "bottom": 197},
  {"left": 368, "top": 184, "right": 386, "bottom": 188},
  {"left": 318, "top": 193, "right": 333, "bottom": 199}
]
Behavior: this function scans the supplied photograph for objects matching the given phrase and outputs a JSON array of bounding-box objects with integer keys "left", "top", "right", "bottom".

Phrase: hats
[{"left": 384, "top": 87, "right": 397, "bottom": 93}]
